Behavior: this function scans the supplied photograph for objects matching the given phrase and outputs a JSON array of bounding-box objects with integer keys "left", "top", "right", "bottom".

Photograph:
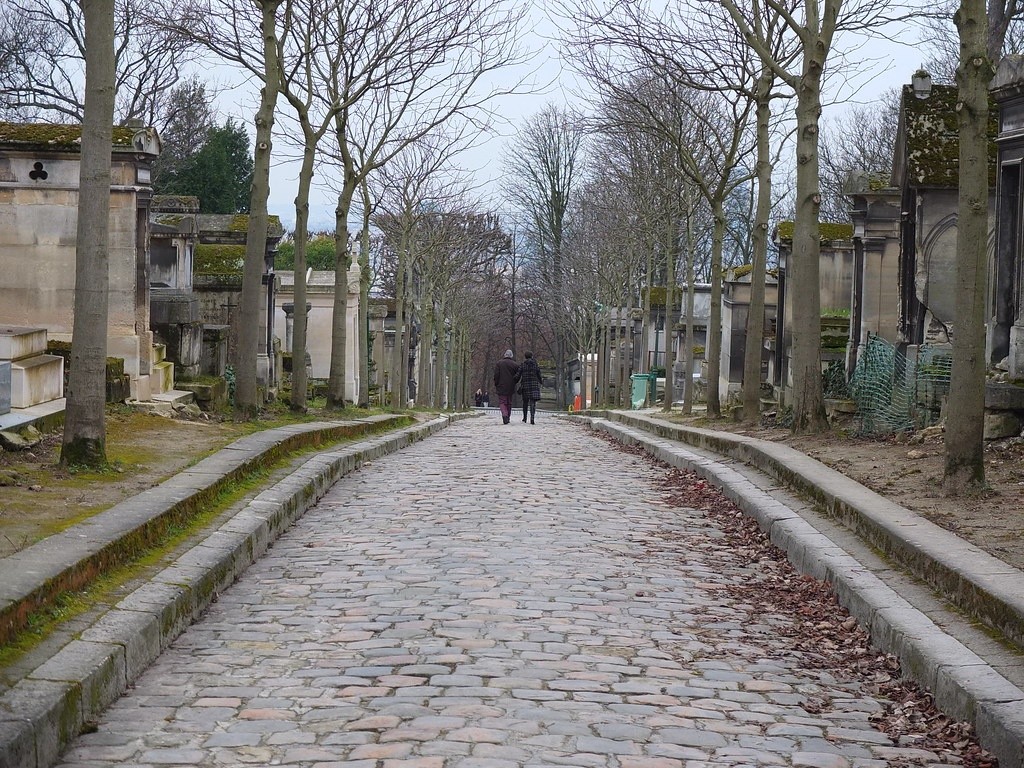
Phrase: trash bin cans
[{"left": 629, "top": 373, "right": 657, "bottom": 410}]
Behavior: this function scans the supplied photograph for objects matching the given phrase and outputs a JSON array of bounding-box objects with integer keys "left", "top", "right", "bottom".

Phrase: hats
[{"left": 505, "top": 350, "right": 513, "bottom": 357}]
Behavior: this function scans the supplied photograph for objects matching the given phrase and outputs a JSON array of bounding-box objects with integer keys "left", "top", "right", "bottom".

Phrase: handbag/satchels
[{"left": 517, "top": 384, "right": 522, "bottom": 395}]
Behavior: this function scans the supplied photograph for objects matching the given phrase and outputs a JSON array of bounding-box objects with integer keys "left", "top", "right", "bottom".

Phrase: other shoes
[
  {"left": 531, "top": 416, "right": 534, "bottom": 424},
  {"left": 522, "top": 415, "right": 527, "bottom": 423},
  {"left": 504, "top": 417, "right": 508, "bottom": 424}
]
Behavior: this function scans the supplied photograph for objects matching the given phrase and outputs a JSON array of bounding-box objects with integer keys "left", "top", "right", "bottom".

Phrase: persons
[
  {"left": 475, "top": 389, "right": 483, "bottom": 407},
  {"left": 494, "top": 350, "right": 519, "bottom": 425},
  {"left": 513, "top": 350, "right": 543, "bottom": 425},
  {"left": 482, "top": 391, "right": 489, "bottom": 407}
]
